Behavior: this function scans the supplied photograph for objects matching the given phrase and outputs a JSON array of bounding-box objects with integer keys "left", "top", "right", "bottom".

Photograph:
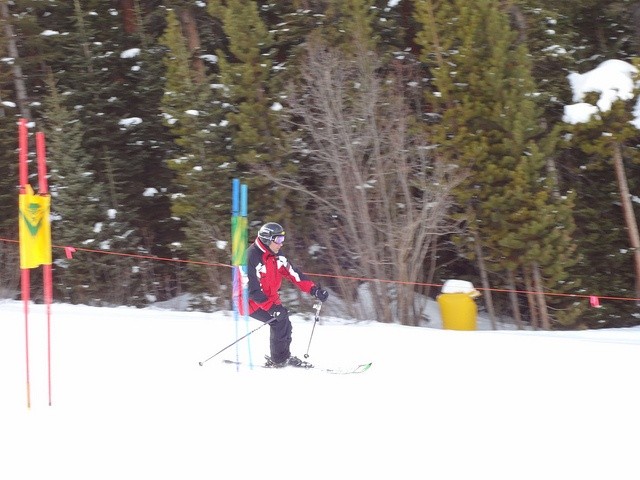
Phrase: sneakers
[
  {"left": 265, "top": 355, "right": 285, "bottom": 369},
  {"left": 288, "top": 355, "right": 303, "bottom": 367}
]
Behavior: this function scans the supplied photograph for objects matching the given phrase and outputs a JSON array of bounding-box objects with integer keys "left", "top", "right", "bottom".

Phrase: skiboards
[{"left": 222, "top": 360, "right": 373, "bottom": 373}]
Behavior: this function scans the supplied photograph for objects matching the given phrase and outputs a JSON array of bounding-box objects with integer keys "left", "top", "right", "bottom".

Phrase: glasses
[{"left": 270, "top": 235, "right": 286, "bottom": 245}]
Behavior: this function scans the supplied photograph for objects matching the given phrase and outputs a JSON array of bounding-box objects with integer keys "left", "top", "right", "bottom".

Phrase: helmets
[{"left": 258, "top": 221, "right": 285, "bottom": 247}]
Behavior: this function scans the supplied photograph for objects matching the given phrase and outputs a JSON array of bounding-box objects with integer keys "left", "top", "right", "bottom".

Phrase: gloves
[
  {"left": 311, "top": 286, "right": 329, "bottom": 302},
  {"left": 268, "top": 305, "right": 287, "bottom": 322}
]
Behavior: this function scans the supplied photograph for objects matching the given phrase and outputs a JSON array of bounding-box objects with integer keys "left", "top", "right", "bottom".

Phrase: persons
[{"left": 231, "top": 222, "right": 330, "bottom": 370}]
